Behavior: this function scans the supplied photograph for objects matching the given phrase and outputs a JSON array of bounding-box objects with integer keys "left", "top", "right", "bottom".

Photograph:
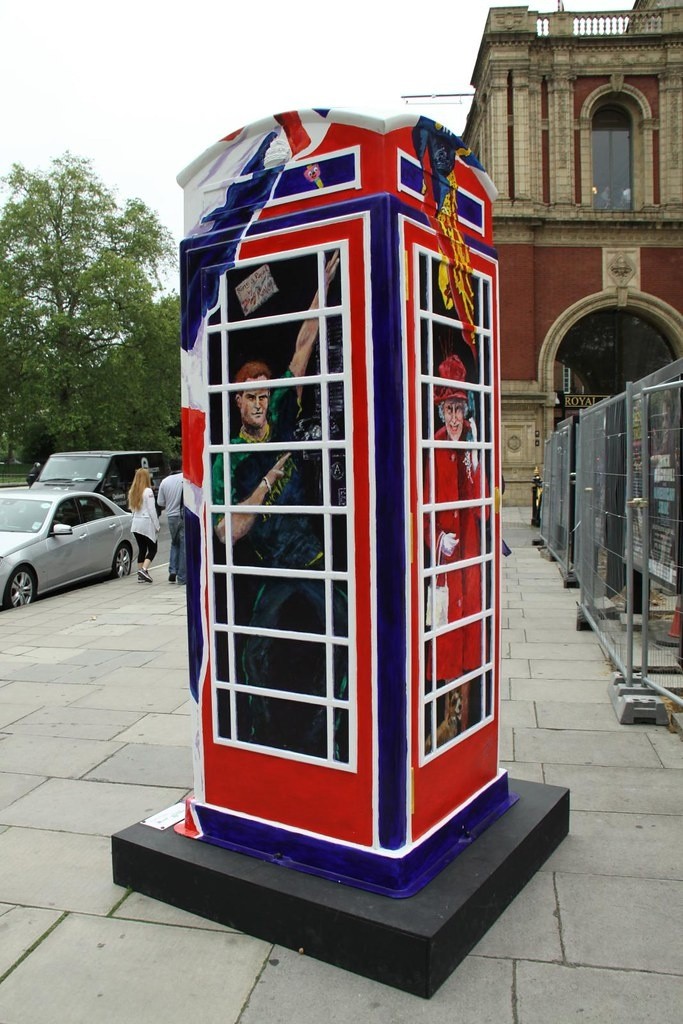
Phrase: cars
[{"left": 0, "top": 490, "right": 139, "bottom": 608}]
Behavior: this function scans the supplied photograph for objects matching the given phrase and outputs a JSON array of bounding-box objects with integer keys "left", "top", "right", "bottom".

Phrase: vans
[{"left": 30, "top": 451, "right": 173, "bottom": 513}]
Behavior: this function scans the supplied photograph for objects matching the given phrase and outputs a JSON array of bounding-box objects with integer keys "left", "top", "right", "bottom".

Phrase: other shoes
[
  {"left": 138, "top": 568, "right": 153, "bottom": 583},
  {"left": 168, "top": 574, "right": 176, "bottom": 582},
  {"left": 138, "top": 575, "right": 146, "bottom": 583}
]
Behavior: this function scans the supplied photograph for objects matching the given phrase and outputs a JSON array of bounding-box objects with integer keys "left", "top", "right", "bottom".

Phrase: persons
[
  {"left": 156, "top": 456, "right": 186, "bottom": 584},
  {"left": 127, "top": 468, "right": 160, "bottom": 583}
]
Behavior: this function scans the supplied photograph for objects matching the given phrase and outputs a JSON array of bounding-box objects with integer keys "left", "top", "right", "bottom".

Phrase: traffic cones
[{"left": 667, "top": 594, "right": 680, "bottom": 637}]
